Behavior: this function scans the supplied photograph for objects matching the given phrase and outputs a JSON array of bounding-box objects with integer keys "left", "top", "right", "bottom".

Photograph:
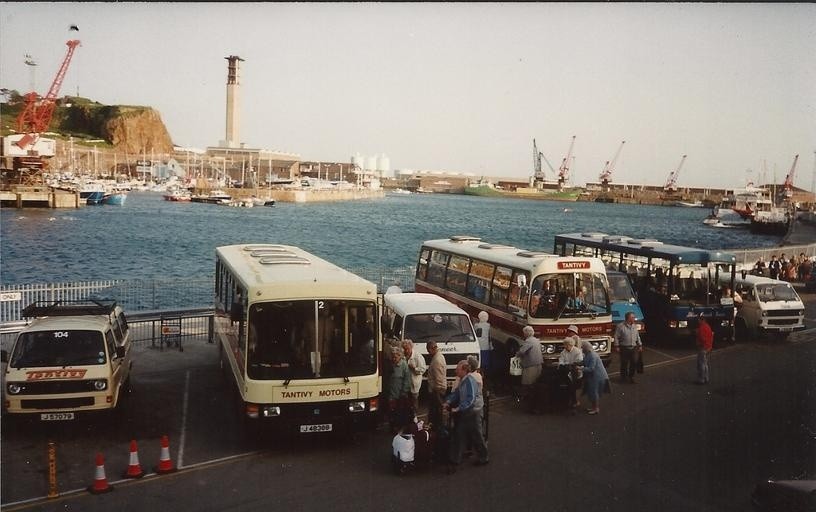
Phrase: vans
[
  {"left": 703, "top": 270, "right": 808, "bottom": 337},
  {"left": 560, "top": 269, "right": 645, "bottom": 347},
  {"left": 380, "top": 285, "right": 484, "bottom": 401},
  {"left": 0, "top": 297, "right": 132, "bottom": 426}
]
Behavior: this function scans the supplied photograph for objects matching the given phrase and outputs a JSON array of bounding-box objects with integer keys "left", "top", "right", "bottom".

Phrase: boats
[
  {"left": 385, "top": 188, "right": 412, "bottom": 198},
  {"left": 42, "top": 135, "right": 294, "bottom": 210},
  {"left": 675, "top": 198, "right": 704, "bottom": 207},
  {"left": 702, "top": 159, "right": 790, "bottom": 236},
  {"left": 417, "top": 178, "right": 619, "bottom": 204}
]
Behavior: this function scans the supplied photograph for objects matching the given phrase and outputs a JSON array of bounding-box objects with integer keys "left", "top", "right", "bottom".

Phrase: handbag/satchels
[
  {"left": 508, "top": 356, "right": 522, "bottom": 376},
  {"left": 636, "top": 350, "right": 645, "bottom": 374},
  {"left": 390, "top": 427, "right": 442, "bottom": 469}
]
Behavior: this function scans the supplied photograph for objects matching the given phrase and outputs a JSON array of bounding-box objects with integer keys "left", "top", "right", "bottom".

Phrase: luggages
[{"left": 431, "top": 407, "right": 459, "bottom": 465}]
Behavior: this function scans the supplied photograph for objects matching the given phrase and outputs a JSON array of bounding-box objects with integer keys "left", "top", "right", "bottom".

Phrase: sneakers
[{"left": 572, "top": 400, "right": 581, "bottom": 407}]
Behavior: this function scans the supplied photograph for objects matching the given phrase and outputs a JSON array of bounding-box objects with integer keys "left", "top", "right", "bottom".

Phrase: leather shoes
[{"left": 586, "top": 407, "right": 599, "bottom": 415}]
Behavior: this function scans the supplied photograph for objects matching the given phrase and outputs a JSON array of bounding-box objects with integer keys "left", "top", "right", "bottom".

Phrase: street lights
[
  {"left": 316, "top": 160, "right": 322, "bottom": 193},
  {"left": 324, "top": 164, "right": 331, "bottom": 181},
  {"left": 338, "top": 164, "right": 343, "bottom": 190}
]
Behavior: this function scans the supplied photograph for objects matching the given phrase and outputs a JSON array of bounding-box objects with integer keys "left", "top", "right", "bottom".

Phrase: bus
[
  {"left": 301, "top": 179, "right": 309, "bottom": 186},
  {"left": 554, "top": 231, "right": 737, "bottom": 347},
  {"left": 213, "top": 243, "right": 383, "bottom": 435},
  {"left": 415, "top": 235, "right": 612, "bottom": 368}
]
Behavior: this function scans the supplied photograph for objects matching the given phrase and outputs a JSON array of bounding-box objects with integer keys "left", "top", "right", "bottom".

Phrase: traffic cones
[
  {"left": 120, "top": 438, "right": 144, "bottom": 479},
  {"left": 157, "top": 433, "right": 175, "bottom": 473},
  {"left": 88, "top": 452, "right": 115, "bottom": 495}
]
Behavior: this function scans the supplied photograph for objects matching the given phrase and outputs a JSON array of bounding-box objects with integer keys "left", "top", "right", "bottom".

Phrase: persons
[
  {"left": 475, "top": 311, "right": 494, "bottom": 373},
  {"left": 754, "top": 253, "right": 816, "bottom": 286},
  {"left": 695, "top": 315, "right": 714, "bottom": 384},
  {"left": 559, "top": 325, "right": 612, "bottom": 414},
  {"left": 542, "top": 280, "right": 554, "bottom": 295},
  {"left": 614, "top": 312, "right": 643, "bottom": 383},
  {"left": 517, "top": 326, "right": 543, "bottom": 401},
  {"left": 387, "top": 340, "right": 489, "bottom": 474},
  {"left": 566, "top": 286, "right": 585, "bottom": 310}
]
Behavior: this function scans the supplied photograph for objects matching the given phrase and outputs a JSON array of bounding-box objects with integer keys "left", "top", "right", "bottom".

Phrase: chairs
[
  {"left": 570, "top": 246, "right": 713, "bottom": 299},
  {"left": 739, "top": 287, "right": 773, "bottom": 299},
  {"left": 419, "top": 259, "right": 539, "bottom": 317}
]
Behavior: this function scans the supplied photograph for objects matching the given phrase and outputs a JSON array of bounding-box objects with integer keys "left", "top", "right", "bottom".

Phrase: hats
[{"left": 567, "top": 325, "right": 579, "bottom": 335}]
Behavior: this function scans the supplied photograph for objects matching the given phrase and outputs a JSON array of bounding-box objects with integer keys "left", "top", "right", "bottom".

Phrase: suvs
[{"left": 804, "top": 261, "right": 816, "bottom": 290}]
